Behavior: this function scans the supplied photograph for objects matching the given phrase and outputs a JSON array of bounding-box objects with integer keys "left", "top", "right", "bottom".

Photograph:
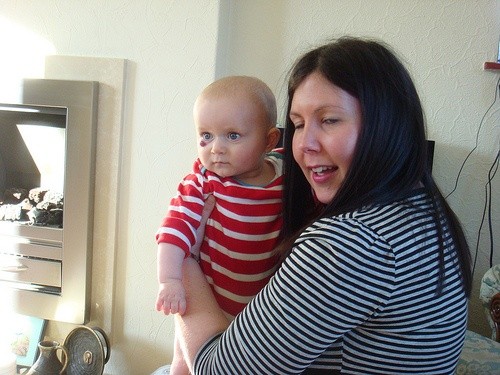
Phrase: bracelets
[{"left": 185, "top": 252, "right": 200, "bottom": 263}]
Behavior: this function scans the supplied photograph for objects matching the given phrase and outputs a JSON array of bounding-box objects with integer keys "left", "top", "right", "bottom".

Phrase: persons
[
  {"left": 155, "top": 75, "right": 327, "bottom": 375},
  {"left": 173, "top": 34, "right": 472, "bottom": 375}
]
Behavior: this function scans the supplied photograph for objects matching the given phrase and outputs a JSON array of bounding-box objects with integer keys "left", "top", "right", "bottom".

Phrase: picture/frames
[{"left": 7, "top": 320, "right": 48, "bottom": 369}]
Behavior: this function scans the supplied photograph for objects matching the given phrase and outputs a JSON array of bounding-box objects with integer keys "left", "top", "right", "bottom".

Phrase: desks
[{"left": 453, "top": 328, "right": 500, "bottom": 375}]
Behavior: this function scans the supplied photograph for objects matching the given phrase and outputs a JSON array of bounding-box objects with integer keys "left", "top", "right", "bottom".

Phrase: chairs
[{"left": 480, "top": 262, "right": 500, "bottom": 342}]
